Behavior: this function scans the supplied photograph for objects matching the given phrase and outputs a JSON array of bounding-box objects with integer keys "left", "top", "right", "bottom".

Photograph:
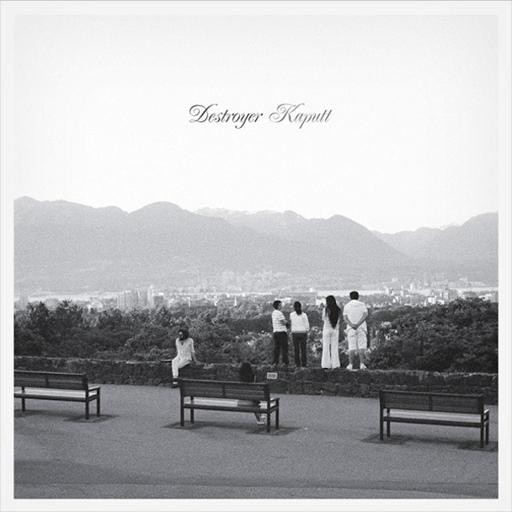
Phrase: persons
[
  {"left": 271, "top": 300, "right": 290, "bottom": 366},
  {"left": 289, "top": 301, "right": 310, "bottom": 367},
  {"left": 321, "top": 295, "right": 341, "bottom": 369},
  {"left": 171, "top": 328, "right": 198, "bottom": 388},
  {"left": 342, "top": 291, "right": 369, "bottom": 370}
]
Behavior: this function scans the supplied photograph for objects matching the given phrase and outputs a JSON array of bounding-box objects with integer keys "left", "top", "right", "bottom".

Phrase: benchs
[
  {"left": 378, "top": 389, "right": 491, "bottom": 448},
  {"left": 14, "top": 370, "right": 101, "bottom": 419},
  {"left": 180, "top": 379, "right": 279, "bottom": 432}
]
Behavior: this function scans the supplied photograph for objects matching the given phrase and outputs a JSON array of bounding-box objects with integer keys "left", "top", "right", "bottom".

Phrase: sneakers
[
  {"left": 172, "top": 384, "right": 178, "bottom": 388},
  {"left": 257, "top": 416, "right": 265, "bottom": 425},
  {"left": 273, "top": 362, "right": 306, "bottom": 367},
  {"left": 360, "top": 363, "right": 367, "bottom": 369},
  {"left": 346, "top": 363, "right": 352, "bottom": 369}
]
